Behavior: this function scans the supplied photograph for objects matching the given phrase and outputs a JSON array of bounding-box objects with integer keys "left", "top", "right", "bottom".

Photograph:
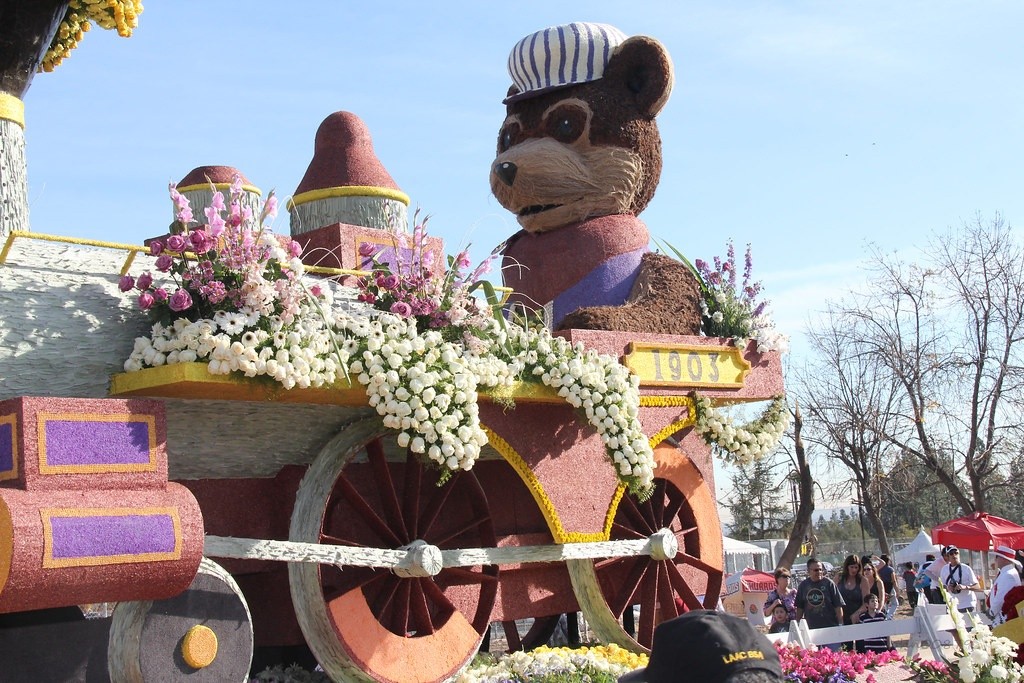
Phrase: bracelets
[
  {"left": 966, "top": 585, "right": 968, "bottom": 590},
  {"left": 839, "top": 624, "right": 844, "bottom": 625}
]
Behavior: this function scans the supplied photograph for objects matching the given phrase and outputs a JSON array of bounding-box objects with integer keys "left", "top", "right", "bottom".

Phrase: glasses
[
  {"left": 948, "top": 552, "right": 959, "bottom": 555},
  {"left": 779, "top": 575, "right": 791, "bottom": 578},
  {"left": 863, "top": 568, "right": 873, "bottom": 571},
  {"left": 809, "top": 568, "right": 823, "bottom": 572}
]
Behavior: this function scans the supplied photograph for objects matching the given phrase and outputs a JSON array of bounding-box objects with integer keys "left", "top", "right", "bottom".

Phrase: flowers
[
  {"left": 125, "top": 167, "right": 789, "bottom": 505},
  {"left": 256, "top": 574, "right": 1024, "bottom": 683}
]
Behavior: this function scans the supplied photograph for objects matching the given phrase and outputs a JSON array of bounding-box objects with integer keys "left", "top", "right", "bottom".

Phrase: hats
[
  {"left": 617, "top": 609, "right": 783, "bottom": 683},
  {"left": 946, "top": 545, "right": 957, "bottom": 554},
  {"left": 989, "top": 546, "right": 1023, "bottom": 567}
]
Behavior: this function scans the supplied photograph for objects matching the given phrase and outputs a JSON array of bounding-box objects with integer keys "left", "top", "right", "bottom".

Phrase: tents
[
  {"left": 722, "top": 536, "right": 769, "bottom": 576},
  {"left": 931, "top": 512, "right": 1024, "bottom": 589},
  {"left": 888, "top": 526, "right": 945, "bottom": 568},
  {"left": 720, "top": 567, "right": 777, "bottom": 625}
]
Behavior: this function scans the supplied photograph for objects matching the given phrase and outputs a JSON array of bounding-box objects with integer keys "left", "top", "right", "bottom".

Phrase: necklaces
[{"left": 848, "top": 582, "right": 850, "bottom": 585}]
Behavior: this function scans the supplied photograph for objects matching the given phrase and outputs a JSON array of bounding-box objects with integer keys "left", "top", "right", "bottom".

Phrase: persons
[
  {"left": 862, "top": 563, "right": 897, "bottom": 652},
  {"left": 832, "top": 555, "right": 871, "bottom": 654},
  {"left": 763, "top": 567, "right": 798, "bottom": 624},
  {"left": 861, "top": 554, "right": 886, "bottom": 571},
  {"left": 878, "top": 555, "right": 899, "bottom": 618},
  {"left": 617, "top": 611, "right": 783, "bottom": 683},
  {"left": 768, "top": 605, "right": 790, "bottom": 633},
  {"left": 901, "top": 547, "right": 951, "bottom": 614},
  {"left": 983, "top": 546, "right": 1024, "bottom": 669},
  {"left": 793, "top": 558, "right": 846, "bottom": 651},
  {"left": 858, "top": 594, "right": 892, "bottom": 655},
  {"left": 990, "top": 563, "right": 1001, "bottom": 585},
  {"left": 939, "top": 545, "right": 980, "bottom": 658}
]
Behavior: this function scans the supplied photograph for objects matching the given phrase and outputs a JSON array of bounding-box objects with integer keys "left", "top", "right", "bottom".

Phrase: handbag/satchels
[
  {"left": 917, "top": 592, "right": 929, "bottom": 607},
  {"left": 913, "top": 569, "right": 931, "bottom": 589}
]
